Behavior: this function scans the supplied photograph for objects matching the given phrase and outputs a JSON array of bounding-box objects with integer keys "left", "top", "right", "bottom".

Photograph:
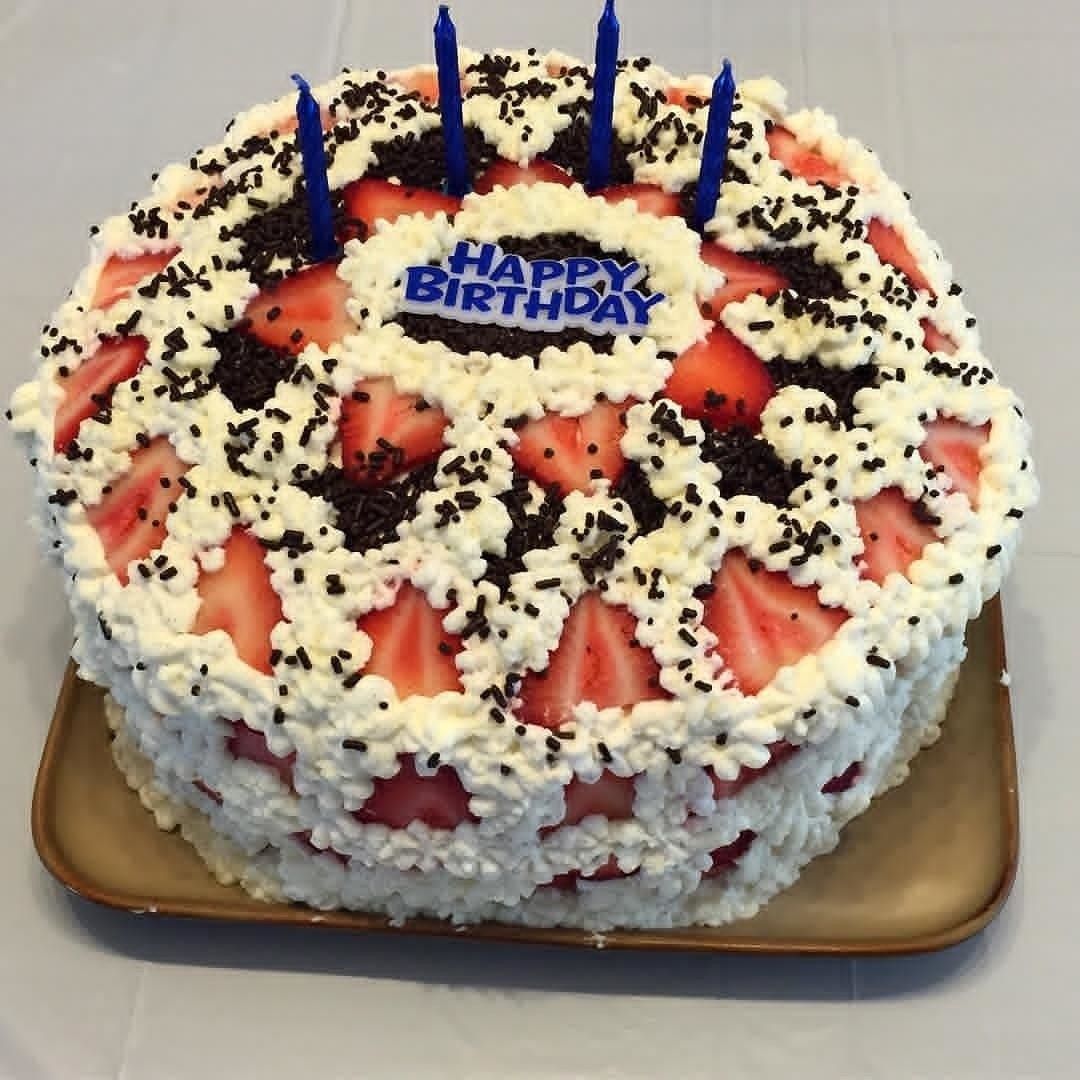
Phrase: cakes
[{"left": 3, "top": 50, "right": 1039, "bottom": 933}]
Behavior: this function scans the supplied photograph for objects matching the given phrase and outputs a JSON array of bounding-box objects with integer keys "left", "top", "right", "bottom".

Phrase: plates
[{"left": 32, "top": 590, "right": 1022, "bottom": 953}]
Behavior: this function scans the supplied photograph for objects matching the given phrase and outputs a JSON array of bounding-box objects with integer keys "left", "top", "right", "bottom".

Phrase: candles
[
  {"left": 694, "top": 57, "right": 736, "bottom": 230},
  {"left": 434, "top": 5, "right": 468, "bottom": 198},
  {"left": 587, "top": 0, "right": 619, "bottom": 193},
  {"left": 291, "top": 73, "right": 337, "bottom": 261}
]
